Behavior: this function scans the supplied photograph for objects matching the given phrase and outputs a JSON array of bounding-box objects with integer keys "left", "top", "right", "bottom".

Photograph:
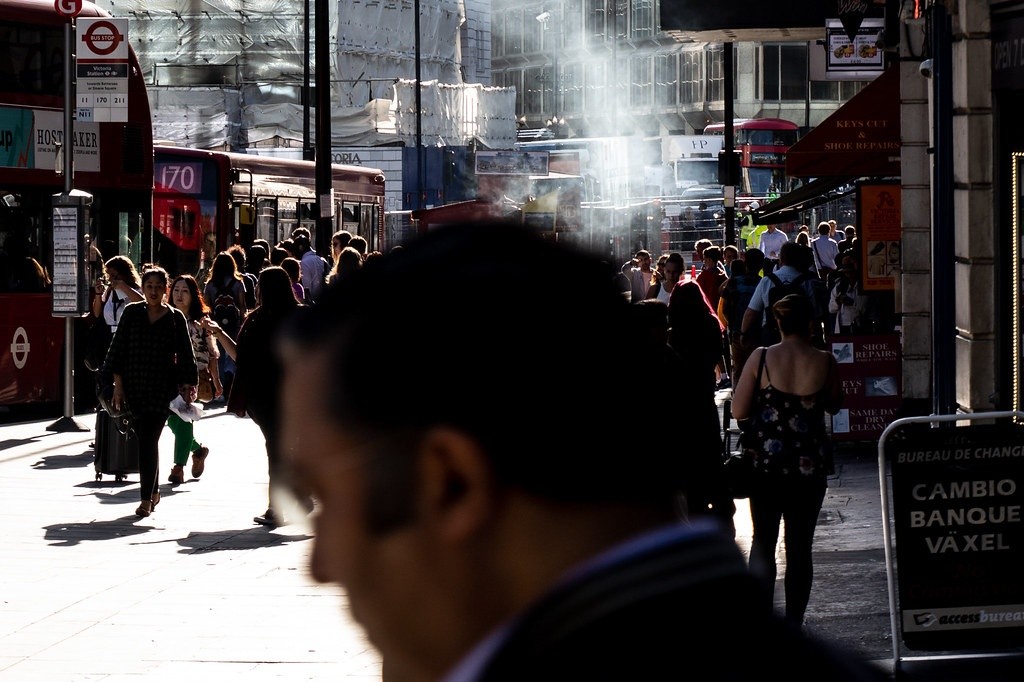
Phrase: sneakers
[
  {"left": 192, "top": 448, "right": 208, "bottom": 478},
  {"left": 716, "top": 378, "right": 731, "bottom": 388},
  {"left": 169, "top": 466, "right": 185, "bottom": 484}
]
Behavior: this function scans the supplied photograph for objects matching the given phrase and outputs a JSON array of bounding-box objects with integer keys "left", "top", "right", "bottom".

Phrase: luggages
[{"left": 95, "top": 408, "right": 139, "bottom": 485}]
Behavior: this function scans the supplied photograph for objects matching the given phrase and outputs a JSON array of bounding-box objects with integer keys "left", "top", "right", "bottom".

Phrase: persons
[{"left": 20, "top": 202, "right": 896, "bottom": 682}]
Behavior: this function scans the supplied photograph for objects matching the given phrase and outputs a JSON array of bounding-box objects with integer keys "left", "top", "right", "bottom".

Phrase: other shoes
[
  {"left": 255, "top": 510, "right": 285, "bottom": 524},
  {"left": 136, "top": 489, "right": 161, "bottom": 516}
]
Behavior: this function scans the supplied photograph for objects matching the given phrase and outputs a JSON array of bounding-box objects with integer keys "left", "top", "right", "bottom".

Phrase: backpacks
[{"left": 766, "top": 272, "right": 818, "bottom": 346}]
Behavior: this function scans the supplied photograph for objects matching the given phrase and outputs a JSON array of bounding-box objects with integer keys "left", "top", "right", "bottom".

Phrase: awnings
[
  {"left": 750, "top": 177, "right": 884, "bottom": 226},
  {"left": 785, "top": 66, "right": 901, "bottom": 177}
]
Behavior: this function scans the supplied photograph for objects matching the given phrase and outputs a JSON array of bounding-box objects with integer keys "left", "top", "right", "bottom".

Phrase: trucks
[{"left": 637, "top": 134, "right": 725, "bottom": 201}]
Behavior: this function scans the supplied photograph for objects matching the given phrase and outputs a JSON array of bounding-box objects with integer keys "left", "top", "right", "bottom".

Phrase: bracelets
[
  {"left": 212, "top": 330, "right": 222, "bottom": 337},
  {"left": 95, "top": 292, "right": 103, "bottom": 295},
  {"left": 740, "top": 333, "right": 747, "bottom": 335}
]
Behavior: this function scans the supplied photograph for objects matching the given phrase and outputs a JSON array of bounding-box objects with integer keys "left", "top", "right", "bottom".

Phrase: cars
[{"left": 677, "top": 183, "right": 740, "bottom": 239}]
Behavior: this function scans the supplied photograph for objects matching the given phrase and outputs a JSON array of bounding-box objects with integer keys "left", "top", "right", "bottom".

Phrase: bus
[
  {"left": 512, "top": 134, "right": 663, "bottom": 208},
  {"left": 0, "top": 0, "right": 158, "bottom": 425},
  {"left": 700, "top": 116, "right": 808, "bottom": 206},
  {"left": 150, "top": 142, "right": 387, "bottom": 328}
]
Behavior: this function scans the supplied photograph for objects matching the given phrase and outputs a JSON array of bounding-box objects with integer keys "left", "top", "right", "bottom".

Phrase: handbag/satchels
[{"left": 722, "top": 451, "right": 749, "bottom": 499}]
